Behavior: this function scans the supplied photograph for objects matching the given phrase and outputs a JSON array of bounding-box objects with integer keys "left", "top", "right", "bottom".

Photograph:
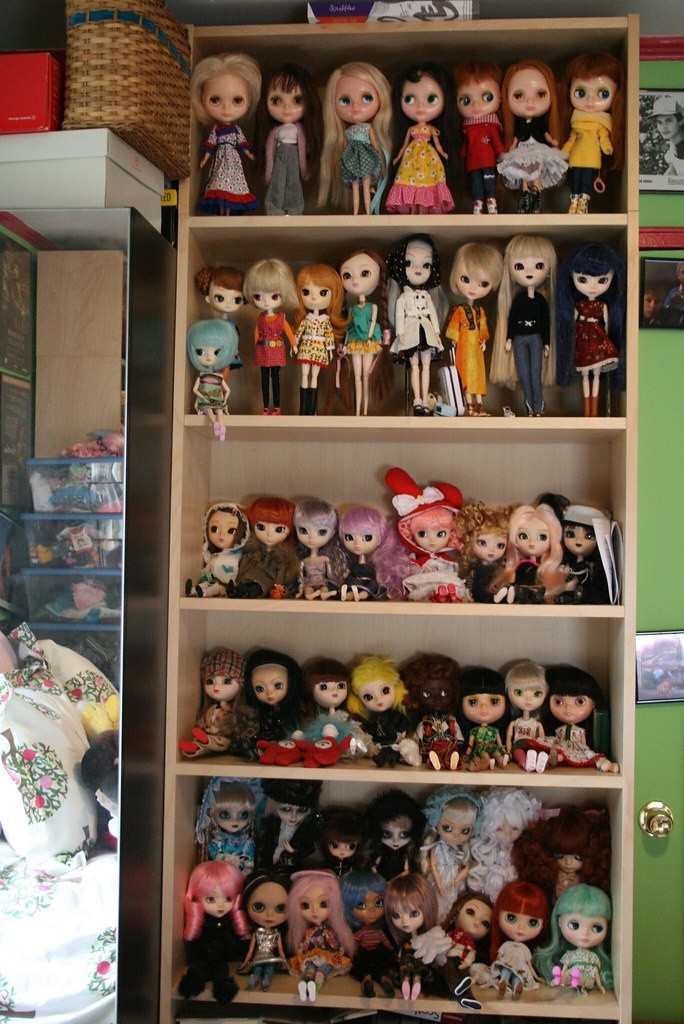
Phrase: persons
[
  {"left": 190, "top": 48, "right": 626, "bottom": 212},
  {"left": 177, "top": 773, "right": 615, "bottom": 1010},
  {"left": 186, "top": 231, "right": 622, "bottom": 441},
  {"left": 645, "top": 97, "right": 684, "bottom": 176},
  {"left": 177, "top": 648, "right": 622, "bottom": 774},
  {"left": 643, "top": 263, "right": 684, "bottom": 329},
  {"left": 184, "top": 463, "right": 624, "bottom": 605}
]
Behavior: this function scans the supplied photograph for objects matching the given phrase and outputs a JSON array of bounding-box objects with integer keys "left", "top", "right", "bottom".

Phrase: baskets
[{"left": 62, "top": 0, "right": 191, "bottom": 181}]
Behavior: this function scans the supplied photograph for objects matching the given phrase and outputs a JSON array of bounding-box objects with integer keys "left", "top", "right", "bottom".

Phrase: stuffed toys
[{"left": 79, "top": 694, "right": 122, "bottom": 852}]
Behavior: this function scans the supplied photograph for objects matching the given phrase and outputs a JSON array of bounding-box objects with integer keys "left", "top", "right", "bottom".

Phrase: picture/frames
[{"left": 638, "top": 89, "right": 684, "bottom": 192}]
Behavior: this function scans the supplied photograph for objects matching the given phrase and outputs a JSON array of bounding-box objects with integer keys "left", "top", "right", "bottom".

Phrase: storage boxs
[
  {"left": 0, "top": 52, "right": 62, "bottom": 135},
  {"left": 21, "top": 567, "right": 123, "bottom": 625},
  {"left": 26, "top": 458, "right": 124, "bottom": 515},
  {"left": 28, "top": 625, "right": 120, "bottom": 688},
  {"left": 19, "top": 513, "right": 124, "bottom": 570},
  {"left": 0, "top": 127, "right": 164, "bottom": 234}
]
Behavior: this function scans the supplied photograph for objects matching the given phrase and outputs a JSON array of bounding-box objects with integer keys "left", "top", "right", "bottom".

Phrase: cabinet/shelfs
[{"left": 158, "top": 9, "right": 641, "bottom": 1024}]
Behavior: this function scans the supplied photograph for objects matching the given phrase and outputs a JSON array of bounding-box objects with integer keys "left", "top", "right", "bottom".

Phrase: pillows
[
  {"left": 33, "top": 638, "right": 121, "bottom": 743},
  {"left": 0, "top": 644, "right": 98, "bottom": 876}
]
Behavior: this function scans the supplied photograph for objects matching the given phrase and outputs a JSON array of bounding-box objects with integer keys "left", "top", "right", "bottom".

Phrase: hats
[{"left": 646, "top": 97, "right": 681, "bottom": 119}]
